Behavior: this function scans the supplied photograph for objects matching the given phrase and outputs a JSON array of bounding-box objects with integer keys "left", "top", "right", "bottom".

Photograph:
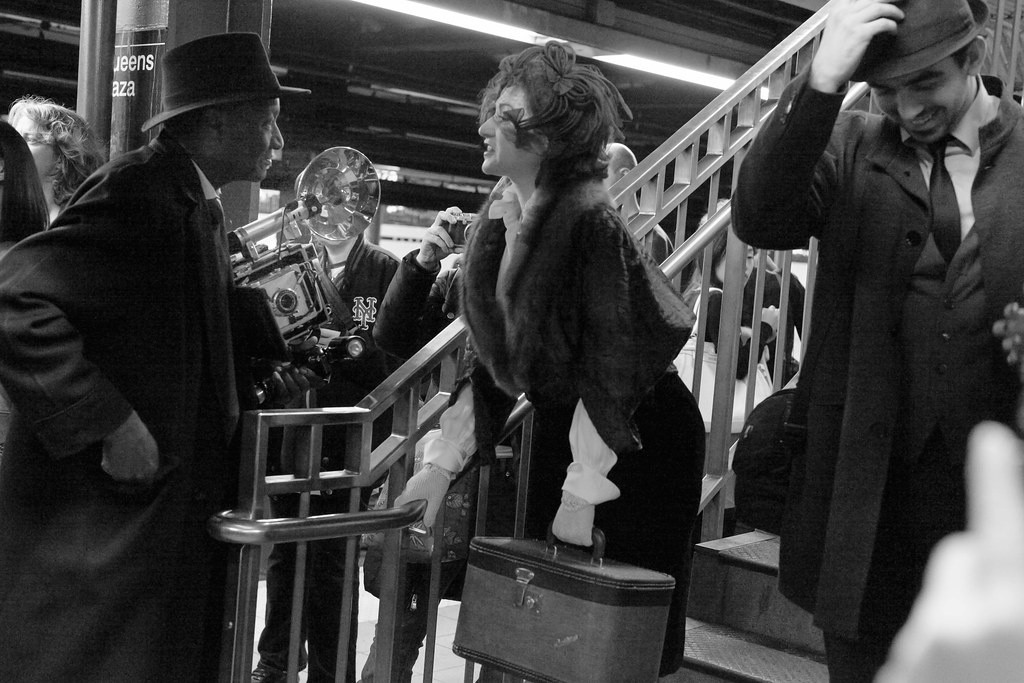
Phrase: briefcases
[{"left": 451, "top": 524, "right": 673, "bottom": 683}]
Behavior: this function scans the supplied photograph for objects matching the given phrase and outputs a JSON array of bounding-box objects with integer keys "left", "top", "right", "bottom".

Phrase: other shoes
[{"left": 248, "top": 666, "right": 301, "bottom": 683}]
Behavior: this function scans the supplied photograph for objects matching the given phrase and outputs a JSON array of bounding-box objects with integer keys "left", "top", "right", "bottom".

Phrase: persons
[
  {"left": 0, "top": 31, "right": 314, "bottom": 683},
  {"left": 602, "top": 142, "right": 673, "bottom": 269},
  {"left": 250, "top": 231, "right": 397, "bottom": 682},
  {"left": 672, "top": 200, "right": 775, "bottom": 477},
  {"left": 0, "top": 120, "right": 53, "bottom": 467},
  {"left": 455, "top": 42, "right": 709, "bottom": 683},
  {"left": 356, "top": 207, "right": 478, "bottom": 683},
  {"left": 730, "top": 0, "right": 1024, "bottom": 681},
  {"left": 777, "top": 265, "right": 807, "bottom": 389},
  {"left": 7, "top": 99, "right": 107, "bottom": 224}
]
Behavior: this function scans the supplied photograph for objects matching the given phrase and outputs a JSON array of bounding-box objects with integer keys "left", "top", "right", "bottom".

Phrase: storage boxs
[{"left": 453, "top": 537, "right": 676, "bottom": 683}]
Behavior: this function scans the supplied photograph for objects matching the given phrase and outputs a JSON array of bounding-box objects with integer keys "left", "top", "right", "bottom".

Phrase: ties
[{"left": 916, "top": 134, "right": 964, "bottom": 267}]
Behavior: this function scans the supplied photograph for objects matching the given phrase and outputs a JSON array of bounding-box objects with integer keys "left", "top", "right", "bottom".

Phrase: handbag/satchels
[
  {"left": 356, "top": 469, "right": 476, "bottom": 567},
  {"left": 731, "top": 388, "right": 809, "bottom": 539}
]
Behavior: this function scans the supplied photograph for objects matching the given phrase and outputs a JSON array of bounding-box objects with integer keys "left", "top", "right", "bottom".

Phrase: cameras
[
  {"left": 226, "top": 147, "right": 382, "bottom": 407},
  {"left": 440, "top": 213, "right": 480, "bottom": 246}
]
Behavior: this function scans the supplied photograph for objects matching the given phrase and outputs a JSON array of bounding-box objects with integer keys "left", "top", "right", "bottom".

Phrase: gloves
[
  {"left": 549, "top": 494, "right": 597, "bottom": 547},
  {"left": 394, "top": 462, "right": 457, "bottom": 530}
]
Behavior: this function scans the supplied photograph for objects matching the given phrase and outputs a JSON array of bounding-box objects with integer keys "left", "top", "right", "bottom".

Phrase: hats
[
  {"left": 142, "top": 31, "right": 312, "bottom": 134},
  {"left": 846, "top": 0, "right": 987, "bottom": 84}
]
